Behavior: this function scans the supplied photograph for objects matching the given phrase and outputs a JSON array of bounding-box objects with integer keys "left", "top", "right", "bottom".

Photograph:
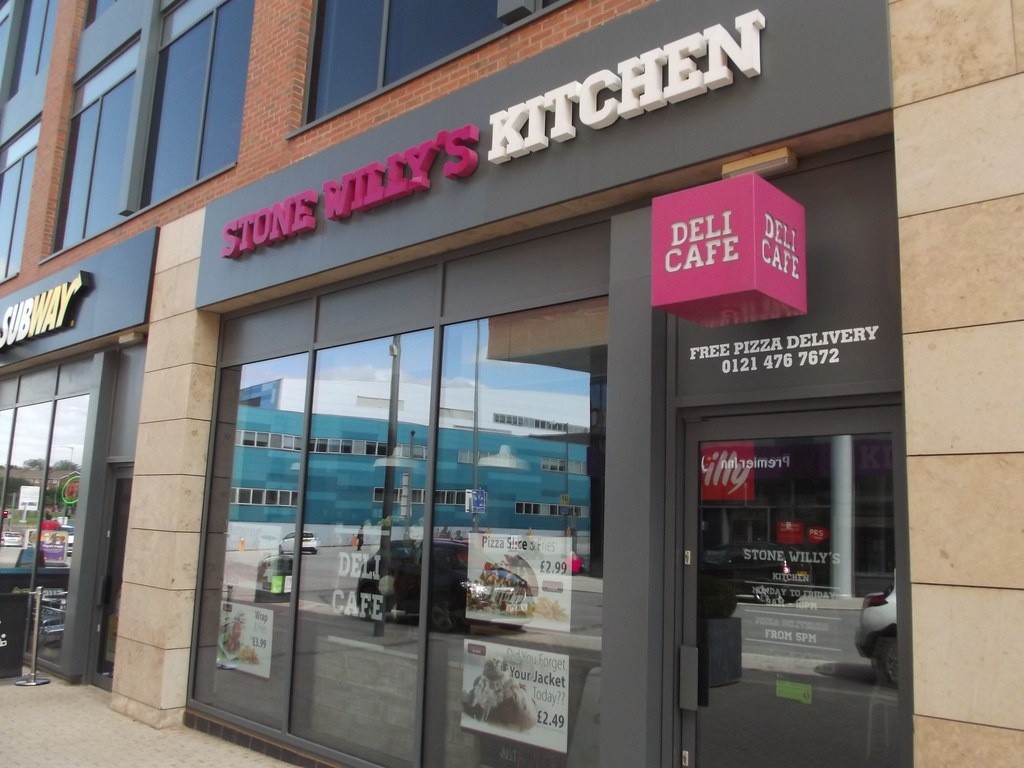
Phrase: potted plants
[{"left": 702, "top": 574, "right": 743, "bottom": 690}]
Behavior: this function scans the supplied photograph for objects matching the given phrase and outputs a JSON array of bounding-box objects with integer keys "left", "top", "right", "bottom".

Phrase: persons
[
  {"left": 441, "top": 526, "right": 450, "bottom": 538},
  {"left": 42, "top": 511, "right": 61, "bottom": 530},
  {"left": 455, "top": 530, "right": 463, "bottom": 540},
  {"left": 357, "top": 525, "right": 364, "bottom": 551}
]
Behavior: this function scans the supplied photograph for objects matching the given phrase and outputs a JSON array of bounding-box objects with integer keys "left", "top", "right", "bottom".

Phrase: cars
[
  {"left": 700, "top": 541, "right": 809, "bottom": 605},
  {"left": 1, "top": 532, "right": 24, "bottom": 547},
  {"left": 278, "top": 532, "right": 318, "bottom": 554},
  {"left": 60, "top": 525, "right": 74, "bottom": 557},
  {"left": 356, "top": 539, "right": 532, "bottom": 633},
  {"left": 854, "top": 567, "right": 896, "bottom": 688}
]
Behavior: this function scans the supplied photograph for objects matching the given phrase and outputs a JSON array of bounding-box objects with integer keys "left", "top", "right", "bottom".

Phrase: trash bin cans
[
  {"left": 15, "top": 546, "right": 70, "bottom": 649},
  {"left": 1, "top": 568, "right": 32, "bottom": 679}
]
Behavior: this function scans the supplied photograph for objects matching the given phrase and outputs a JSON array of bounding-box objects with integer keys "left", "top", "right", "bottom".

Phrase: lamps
[
  {"left": 479, "top": 445, "right": 532, "bottom": 474},
  {"left": 374, "top": 446, "right": 420, "bottom": 469},
  {"left": 721, "top": 146, "right": 798, "bottom": 180},
  {"left": 118, "top": 331, "right": 144, "bottom": 344}
]
